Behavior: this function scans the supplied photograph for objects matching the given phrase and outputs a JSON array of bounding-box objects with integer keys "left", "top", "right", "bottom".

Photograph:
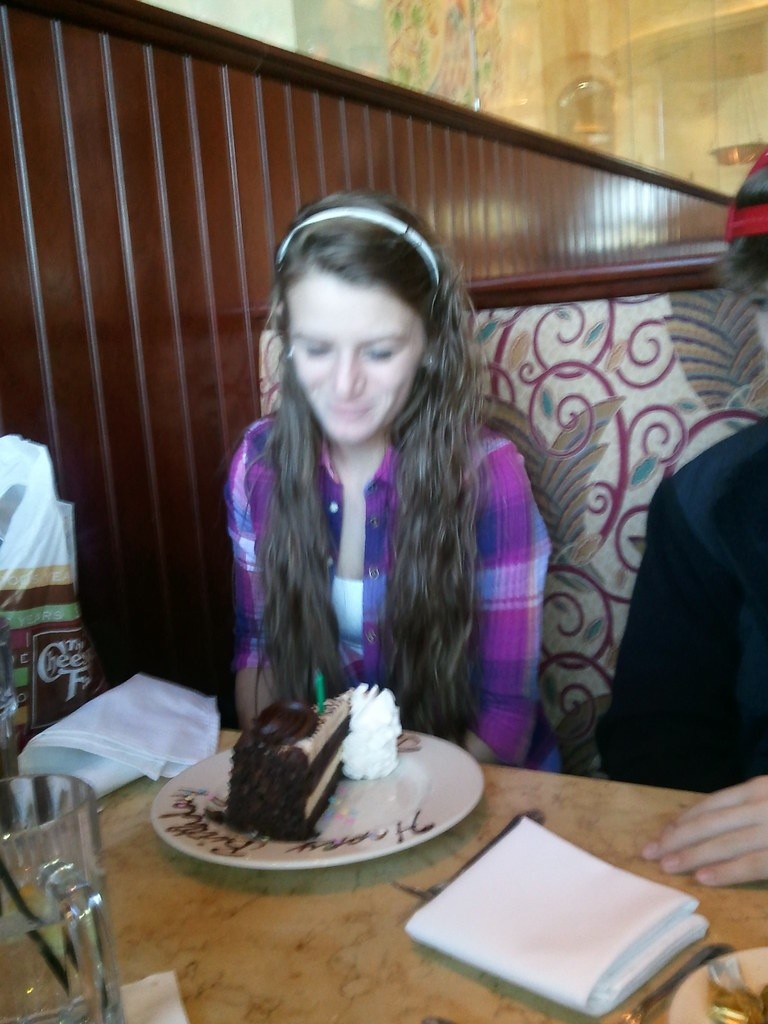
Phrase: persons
[
  {"left": 223, "top": 188, "right": 565, "bottom": 776},
  {"left": 595, "top": 143, "right": 768, "bottom": 887}
]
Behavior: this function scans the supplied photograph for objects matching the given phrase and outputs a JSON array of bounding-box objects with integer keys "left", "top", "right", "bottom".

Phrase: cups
[{"left": 1, "top": 772, "right": 122, "bottom": 1023}]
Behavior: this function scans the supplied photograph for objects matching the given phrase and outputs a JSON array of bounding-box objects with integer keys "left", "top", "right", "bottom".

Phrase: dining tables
[{"left": 2, "top": 728, "right": 768, "bottom": 1023}]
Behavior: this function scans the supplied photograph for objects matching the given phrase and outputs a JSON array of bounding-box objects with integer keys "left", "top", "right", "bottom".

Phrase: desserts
[{"left": 225, "top": 688, "right": 353, "bottom": 843}]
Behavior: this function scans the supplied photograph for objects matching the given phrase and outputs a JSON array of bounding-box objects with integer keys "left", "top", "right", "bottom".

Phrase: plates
[
  {"left": 150, "top": 714, "right": 484, "bottom": 872},
  {"left": 668, "top": 945, "right": 767, "bottom": 1023}
]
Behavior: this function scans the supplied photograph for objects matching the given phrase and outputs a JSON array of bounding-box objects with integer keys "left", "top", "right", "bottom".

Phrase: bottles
[{"left": 1, "top": 615, "right": 23, "bottom": 784}]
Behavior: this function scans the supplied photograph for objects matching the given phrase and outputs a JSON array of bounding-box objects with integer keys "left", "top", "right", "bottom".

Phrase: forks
[{"left": 389, "top": 808, "right": 542, "bottom": 907}]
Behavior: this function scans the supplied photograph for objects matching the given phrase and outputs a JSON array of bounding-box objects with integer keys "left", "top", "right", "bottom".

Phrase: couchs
[{"left": 234, "top": 247, "right": 763, "bottom": 782}]
